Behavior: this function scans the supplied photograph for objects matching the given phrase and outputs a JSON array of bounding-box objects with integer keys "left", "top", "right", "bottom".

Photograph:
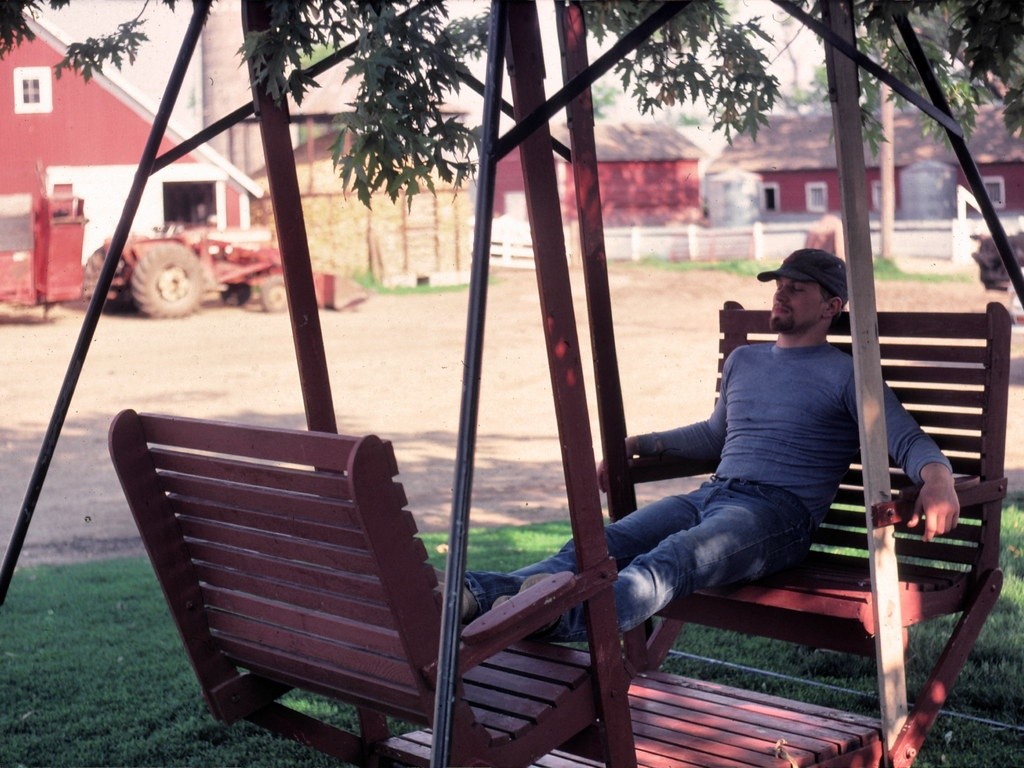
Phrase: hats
[{"left": 758, "top": 249, "right": 848, "bottom": 308}]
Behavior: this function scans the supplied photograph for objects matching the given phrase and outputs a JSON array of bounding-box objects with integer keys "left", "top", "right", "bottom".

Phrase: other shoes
[
  {"left": 433, "top": 568, "right": 478, "bottom": 623},
  {"left": 491, "top": 573, "right": 552, "bottom": 610}
]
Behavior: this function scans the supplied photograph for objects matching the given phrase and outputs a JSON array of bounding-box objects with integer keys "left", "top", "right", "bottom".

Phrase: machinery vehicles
[{"left": 5, "top": 186, "right": 368, "bottom": 320}]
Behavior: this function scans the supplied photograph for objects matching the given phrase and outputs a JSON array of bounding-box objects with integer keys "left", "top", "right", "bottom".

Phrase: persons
[{"left": 462, "top": 248, "right": 959, "bottom": 643}]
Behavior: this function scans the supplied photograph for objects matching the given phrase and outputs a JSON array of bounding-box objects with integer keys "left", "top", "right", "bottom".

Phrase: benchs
[
  {"left": 632, "top": 303, "right": 1012, "bottom": 656},
  {"left": 106, "top": 409, "right": 887, "bottom": 768}
]
